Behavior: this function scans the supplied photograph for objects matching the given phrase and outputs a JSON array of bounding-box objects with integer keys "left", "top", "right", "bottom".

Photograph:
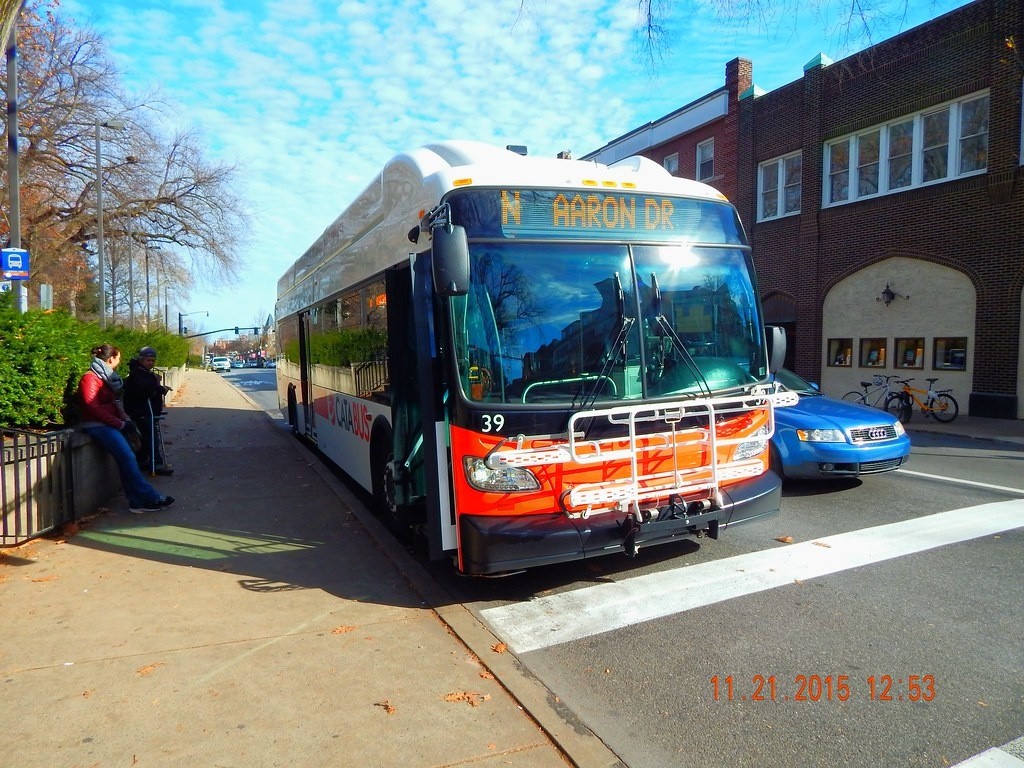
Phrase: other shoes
[
  {"left": 129, "top": 503, "right": 161, "bottom": 513},
  {"left": 155, "top": 464, "right": 173, "bottom": 474},
  {"left": 156, "top": 496, "right": 175, "bottom": 506}
]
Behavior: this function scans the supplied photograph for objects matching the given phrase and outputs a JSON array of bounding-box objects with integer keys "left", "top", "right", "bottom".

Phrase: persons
[
  {"left": 76, "top": 344, "right": 175, "bottom": 513},
  {"left": 123, "top": 346, "right": 174, "bottom": 477}
]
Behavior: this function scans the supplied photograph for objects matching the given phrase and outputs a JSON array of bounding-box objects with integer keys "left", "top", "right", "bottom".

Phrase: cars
[
  {"left": 234, "top": 361, "right": 243, "bottom": 368},
  {"left": 769, "top": 368, "right": 910, "bottom": 490},
  {"left": 231, "top": 362, "right": 276, "bottom": 368}
]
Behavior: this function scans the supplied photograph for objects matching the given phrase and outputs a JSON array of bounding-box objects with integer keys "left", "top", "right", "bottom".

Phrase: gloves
[
  {"left": 127, "top": 427, "right": 142, "bottom": 451},
  {"left": 121, "top": 420, "right": 136, "bottom": 433}
]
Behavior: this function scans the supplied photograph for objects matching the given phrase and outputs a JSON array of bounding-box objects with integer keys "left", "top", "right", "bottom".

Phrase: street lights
[
  {"left": 145, "top": 244, "right": 161, "bottom": 332},
  {"left": 95, "top": 118, "right": 127, "bottom": 331},
  {"left": 165, "top": 287, "right": 175, "bottom": 334}
]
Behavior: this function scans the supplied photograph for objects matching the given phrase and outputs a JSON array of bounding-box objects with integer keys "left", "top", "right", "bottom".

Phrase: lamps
[{"left": 882, "top": 282, "right": 895, "bottom": 307}]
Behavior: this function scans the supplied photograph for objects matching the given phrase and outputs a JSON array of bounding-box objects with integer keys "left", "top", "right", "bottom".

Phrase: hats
[{"left": 140, "top": 347, "right": 157, "bottom": 357}]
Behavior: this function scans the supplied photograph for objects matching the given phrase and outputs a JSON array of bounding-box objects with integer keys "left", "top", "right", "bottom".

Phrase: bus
[{"left": 275, "top": 142, "right": 786, "bottom": 577}]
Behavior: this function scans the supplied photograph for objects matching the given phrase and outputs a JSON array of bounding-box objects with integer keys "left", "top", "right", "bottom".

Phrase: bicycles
[
  {"left": 884, "top": 378, "right": 959, "bottom": 425},
  {"left": 841, "top": 375, "right": 904, "bottom": 420}
]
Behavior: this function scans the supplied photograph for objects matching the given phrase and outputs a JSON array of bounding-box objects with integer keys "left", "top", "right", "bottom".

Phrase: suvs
[{"left": 211, "top": 357, "right": 231, "bottom": 372}]
